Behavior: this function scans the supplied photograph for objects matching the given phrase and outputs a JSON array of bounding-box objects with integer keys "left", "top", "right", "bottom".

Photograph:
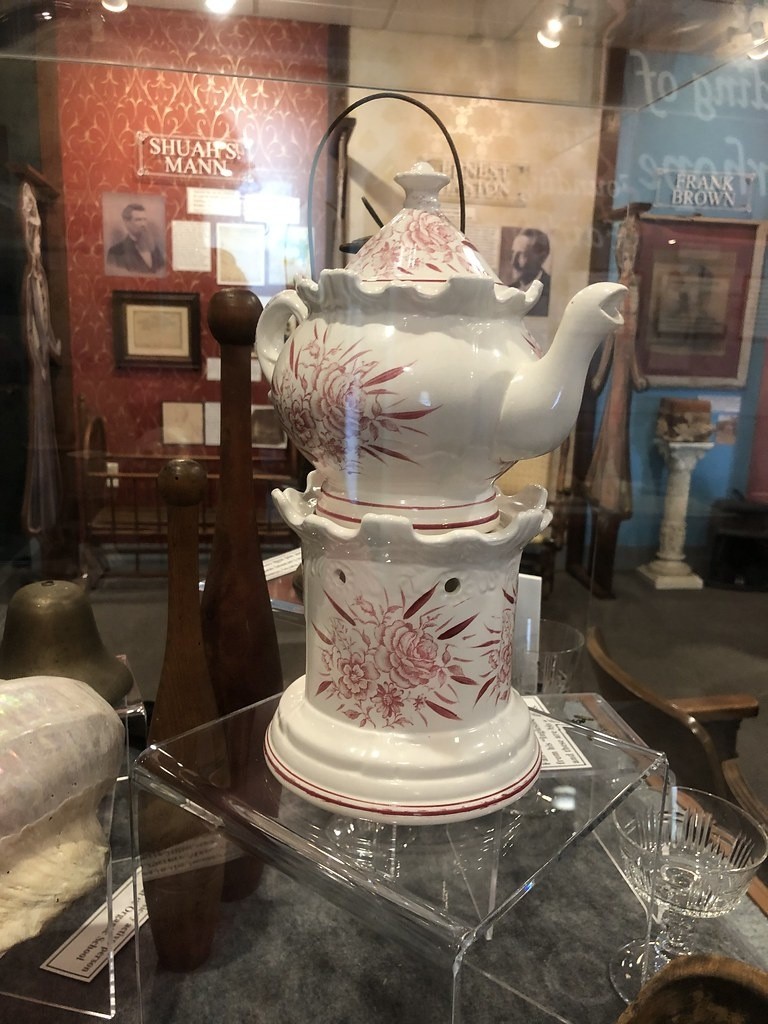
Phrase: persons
[
  {"left": 501, "top": 227, "right": 550, "bottom": 317},
  {"left": 23, "top": 185, "right": 67, "bottom": 535},
  {"left": 107, "top": 204, "right": 164, "bottom": 273},
  {"left": 663, "top": 262, "right": 727, "bottom": 339}
]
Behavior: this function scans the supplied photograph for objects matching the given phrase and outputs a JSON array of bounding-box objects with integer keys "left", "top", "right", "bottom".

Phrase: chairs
[{"left": 585, "top": 626, "right": 768, "bottom": 889}]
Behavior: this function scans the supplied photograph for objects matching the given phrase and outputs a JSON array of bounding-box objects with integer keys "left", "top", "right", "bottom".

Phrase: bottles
[{"left": 509, "top": 617, "right": 586, "bottom": 718}]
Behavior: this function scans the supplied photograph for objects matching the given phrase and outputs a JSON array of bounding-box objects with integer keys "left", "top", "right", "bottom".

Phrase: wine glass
[{"left": 609, "top": 784, "right": 767, "bottom": 1012}]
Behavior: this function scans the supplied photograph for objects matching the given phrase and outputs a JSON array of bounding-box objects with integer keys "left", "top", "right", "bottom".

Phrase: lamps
[
  {"left": 537, "top": 0, "right": 589, "bottom": 49},
  {"left": 101, "top": 0, "right": 128, "bottom": 13}
]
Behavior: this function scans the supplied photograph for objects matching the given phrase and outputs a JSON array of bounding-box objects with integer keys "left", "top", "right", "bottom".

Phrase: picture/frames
[{"left": 634, "top": 213, "right": 768, "bottom": 389}]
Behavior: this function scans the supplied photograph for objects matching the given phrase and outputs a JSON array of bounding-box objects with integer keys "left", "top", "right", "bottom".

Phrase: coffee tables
[{"left": 131, "top": 692, "right": 672, "bottom": 1024}]
